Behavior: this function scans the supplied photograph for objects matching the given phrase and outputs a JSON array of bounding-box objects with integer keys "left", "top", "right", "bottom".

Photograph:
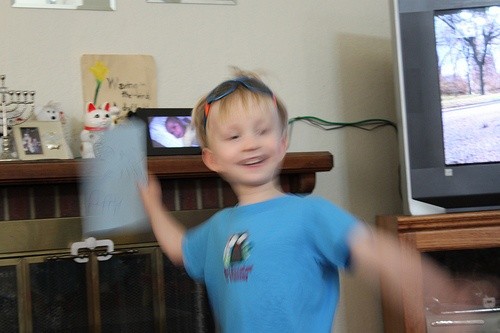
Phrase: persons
[
  {"left": 23, "top": 133, "right": 42, "bottom": 154},
  {"left": 165, "top": 117, "right": 199, "bottom": 146},
  {"left": 135, "top": 65, "right": 499, "bottom": 332}
]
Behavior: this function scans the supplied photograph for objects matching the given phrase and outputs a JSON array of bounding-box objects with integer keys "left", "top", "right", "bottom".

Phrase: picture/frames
[{"left": 136, "top": 108, "right": 203, "bottom": 155}]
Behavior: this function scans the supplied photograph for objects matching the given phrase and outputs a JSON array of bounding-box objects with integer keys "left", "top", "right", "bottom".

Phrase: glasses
[{"left": 204, "top": 77, "right": 278, "bottom": 129}]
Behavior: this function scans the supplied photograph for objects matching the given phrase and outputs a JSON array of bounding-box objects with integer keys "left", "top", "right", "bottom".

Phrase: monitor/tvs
[{"left": 390, "top": 0, "right": 500, "bottom": 217}]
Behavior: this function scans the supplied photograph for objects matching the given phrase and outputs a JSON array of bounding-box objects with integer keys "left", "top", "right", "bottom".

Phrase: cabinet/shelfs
[
  {"left": 375, "top": 210, "right": 500, "bottom": 333},
  {"left": 0, "top": 149, "right": 335, "bottom": 333}
]
